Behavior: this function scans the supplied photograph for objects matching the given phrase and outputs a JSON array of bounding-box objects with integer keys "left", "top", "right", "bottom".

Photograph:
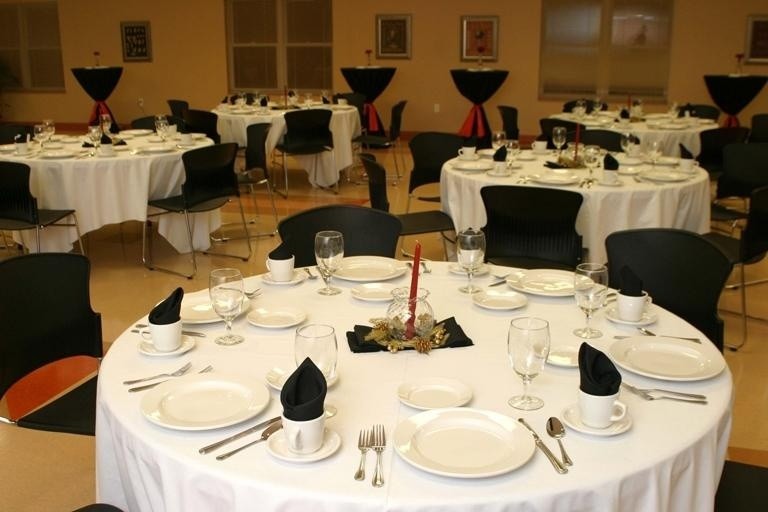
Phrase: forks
[
  {"left": 128, "top": 366, "right": 214, "bottom": 391},
  {"left": 123, "top": 362, "right": 195, "bottom": 385},
  {"left": 622, "top": 382, "right": 710, "bottom": 399},
  {"left": 215, "top": 422, "right": 283, "bottom": 461},
  {"left": 353, "top": 425, "right": 386, "bottom": 489},
  {"left": 631, "top": 390, "right": 708, "bottom": 405}
]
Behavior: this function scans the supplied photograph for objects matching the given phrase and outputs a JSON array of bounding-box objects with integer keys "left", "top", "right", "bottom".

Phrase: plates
[
  {"left": 488, "top": 170, "right": 511, "bottom": 177},
  {"left": 41, "top": 150, "right": 77, "bottom": 158},
  {"left": 265, "top": 365, "right": 342, "bottom": 393},
  {"left": 597, "top": 182, "right": 625, "bottom": 187},
  {"left": 479, "top": 149, "right": 506, "bottom": 156},
  {"left": 562, "top": 403, "right": 634, "bottom": 436},
  {"left": 527, "top": 170, "right": 580, "bottom": 186},
  {"left": 155, "top": 290, "right": 249, "bottom": 325},
  {"left": 473, "top": 288, "right": 529, "bottom": 309},
  {"left": 392, "top": 407, "right": 535, "bottom": 478},
  {"left": 246, "top": 306, "right": 307, "bottom": 329},
  {"left": 267, "top": 425, "right": 341, "bottom": 464},
  {"left": 506, "top": 268, "right": 594, "bottom": 296},
  {"left": 609, "top": 309, "right": 659, "bottom": 326},
  {"left": 529, "top": 151, "right": 552, "bottom": 156},
  {"left": 261, "top": 271, "right": 306, "bottom": 287},
  {"left": 137, "top": 334, "right": 196, "bottom": 356},
  {"left": 547, "top": 349, "right": 586, "bottom": 367},
  {"left": 351, "top": 283, "right": 403, "bottom": 302},
  {"left": 325, "top": 255, "right": 408, "bottom": 282},
  {"left": 458, "top": 156, "right": 480, "bottom": 161},
  {"left": 451, "top": 162, "right": 494, "bottom": 171},
  {"left": 141, "top": 372, "right": 271, "bottom": 431},
  {"left": 0, "top": 144, "right": 29, "bottom": 151},
  {"left": 609, "top": 336, "right": 725, "bottom": 381},
  {"left": 396, "top": 377, "right": 474, "bottom": 410},
  {"left": 639, "top": 171, "right": 688, "bottom": 181}
]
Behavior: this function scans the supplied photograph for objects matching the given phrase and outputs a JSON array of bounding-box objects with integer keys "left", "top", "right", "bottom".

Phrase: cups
[
  {"left": 264, "top": 255, "right": 295, "bottom": 282},
  {"left": 139, "top": 316, "right": 183, "bottom": 350},
  {"left": 679, "top": 159, "right": 699, "bottom": 174},
  {"left": 281, "top": 413, "right": 326, "bottom": 455},
  {"left": 531, "top": 142, "right": 547, "bottom": 152},
  {"left": 493, "top": 162, "right": 510, "bottom": 174},
  {"left": 294, "top": 324, "right": 338, "bottom": 380},
  {"left": 616, "top": 289, "right": 652, "bottom": 322},
  {"left": 603, "top": 171, "right": 621, "bottom": 184},
  {"left": 578, "top": 386, "right": 626, "bottom": 429},
  {"left": 15, "top": 143, "right": 29, "bottom": 153},
  {"left": 458, "top": 146, "right": 478, "bottom": 158}
]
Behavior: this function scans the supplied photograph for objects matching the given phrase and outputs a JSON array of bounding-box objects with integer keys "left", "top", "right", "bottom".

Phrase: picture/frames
[
  {"left": 376, "top": 14, "right": 411, "bottom": 59},
  {"left": 120, "top": 21, "right": 151, "bottom": 62},
  {"left": 745, "top": 15, "right": 768, "bottom": 65},
  {"left": 461, "top": 15, "right": 497, "bottom": 62}
]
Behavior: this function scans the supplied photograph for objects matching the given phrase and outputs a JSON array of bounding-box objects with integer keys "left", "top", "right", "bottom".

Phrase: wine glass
[
  {"left": 216, "top": 88, "right": 356, "bottom": 115},
  {"left": 582, "top": 144, "right": 601, "bottom": 187},
  {"left": 100, "top": 114, "right": 111, "bottom": 131},
  {"left": 553, "top": 127, "right": 567, "bottom": 158},
  {"left": 647, "top": 142, "right": 664, "bottom": 173},
  {"left": 572, "top": 94, "right": 682, "bottom": 127},
  {"left": 491, "top": 131, "right": 507, "bottom": 151},
  {"left": 620, "top": 132, "right": 635, "bottom": 161},
  {"left": 456, "top": 231, "right": 484, "bottom": 293},
  {"left": 315, "top": 230, "right": 343, "bottom": 295},
  {"left": 209, "top": 268, "right": 245, "bottom": 345},
  {"left": 573, "top": 262, "right": 608, "bottom": 338},
  {"left": 155, "top": 121, "right": 168, "bottom": 143},
  {"left": 88, "top": 127, "right": 102, "bottom": 158},
  {"left": 507, "top": 316, "right": 550, "bottom": 409},
  {"left": 42, "top": 120, "right": 56, "bottom": 146},
  {"left": 34, "top": 125, "right": 49, "bottom": 153}
]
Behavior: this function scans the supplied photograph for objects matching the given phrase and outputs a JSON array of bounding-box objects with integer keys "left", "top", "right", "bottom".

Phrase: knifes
[
  {"left": 199, "top": 416, "right": 282, "bottom": 453},
  {"left": 131, "top": 323, "right": 208, "bottom": 338},
  {"left": 516, "top": 417, "right": 568, "bottom": 475}
]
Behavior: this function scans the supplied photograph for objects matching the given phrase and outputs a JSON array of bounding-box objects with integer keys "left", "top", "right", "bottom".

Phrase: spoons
[{"left": 546, "top": 416, "right": 574, "bottom": 466}]
